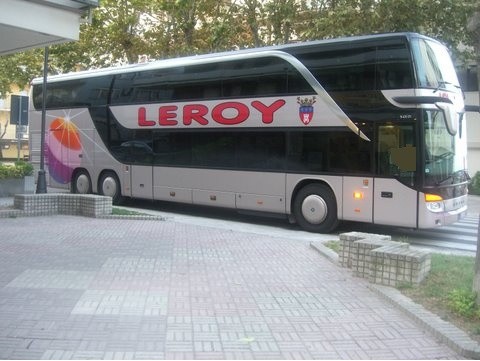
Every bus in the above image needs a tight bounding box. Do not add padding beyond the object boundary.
[28,32,480,234]
